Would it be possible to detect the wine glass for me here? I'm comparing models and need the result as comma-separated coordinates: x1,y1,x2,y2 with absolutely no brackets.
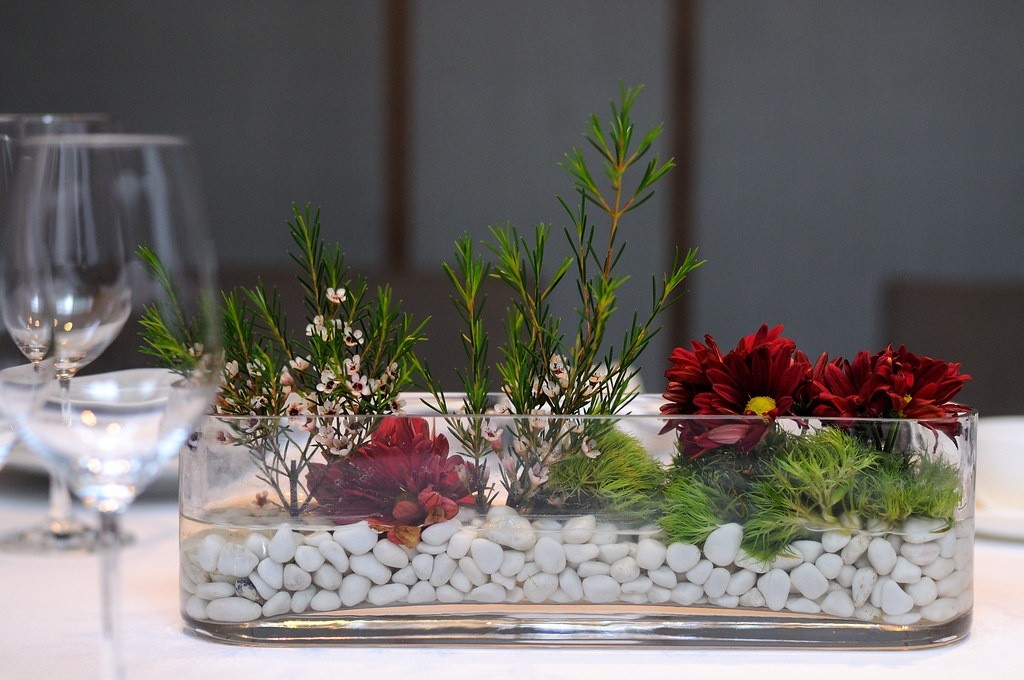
0,115,139,554
0,136,225,680
